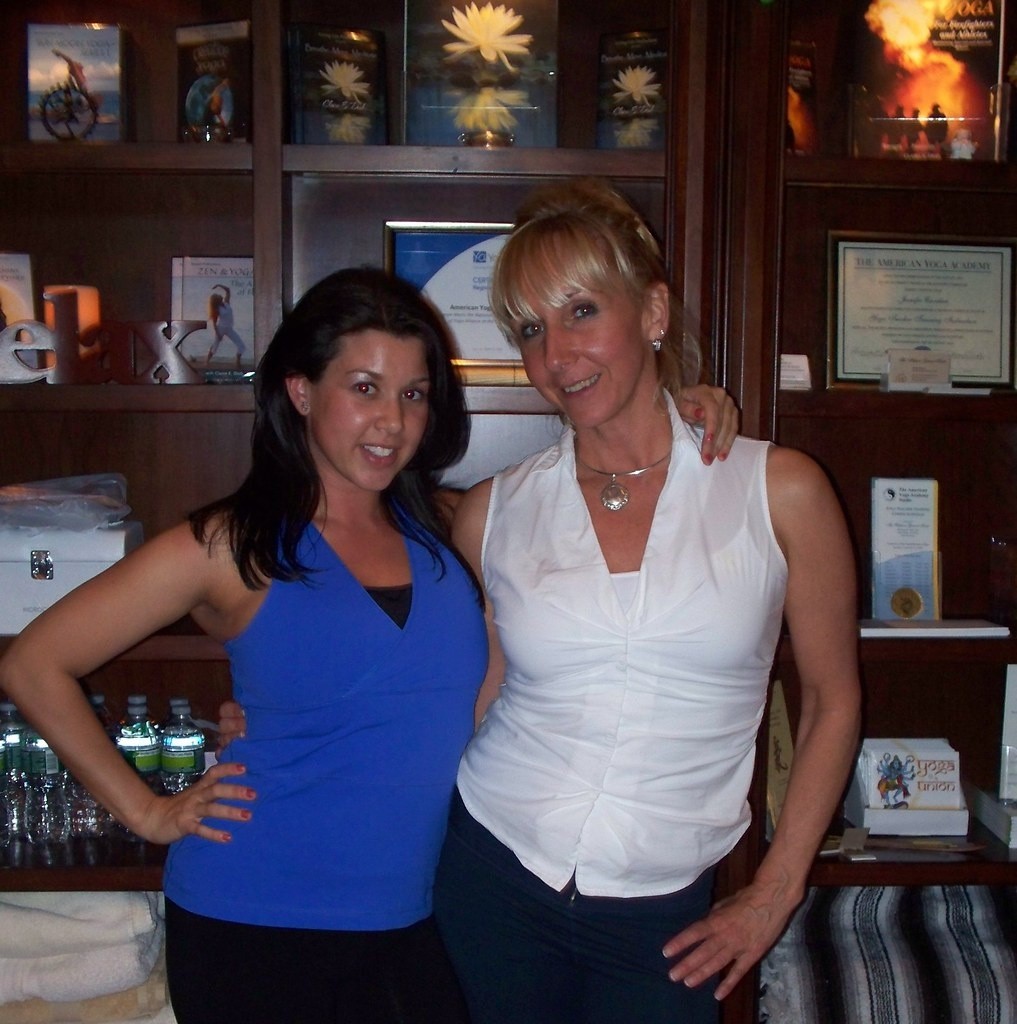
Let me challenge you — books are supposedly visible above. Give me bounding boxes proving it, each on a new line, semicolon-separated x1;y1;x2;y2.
169;257;255;362
844;0;1004;157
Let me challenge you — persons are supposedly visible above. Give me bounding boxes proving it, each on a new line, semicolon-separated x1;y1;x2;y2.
202;281;245;363
219;170;865;1024
1;260;739;1024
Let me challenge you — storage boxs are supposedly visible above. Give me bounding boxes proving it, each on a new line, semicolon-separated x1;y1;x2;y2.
842;765;969;836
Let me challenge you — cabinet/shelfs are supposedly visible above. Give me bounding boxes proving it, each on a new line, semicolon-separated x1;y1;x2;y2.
0;0;1017;885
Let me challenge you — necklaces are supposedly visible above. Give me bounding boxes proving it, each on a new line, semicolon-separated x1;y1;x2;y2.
574;448;672;508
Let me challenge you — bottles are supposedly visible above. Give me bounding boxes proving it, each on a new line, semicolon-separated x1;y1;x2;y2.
0;694;206;845
